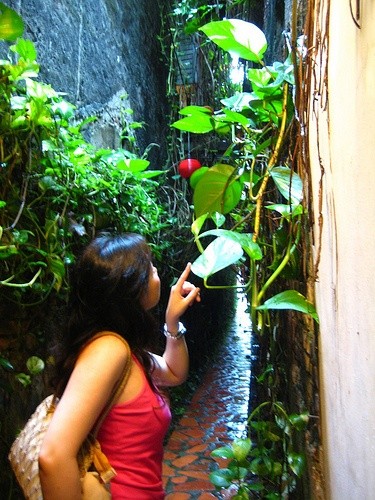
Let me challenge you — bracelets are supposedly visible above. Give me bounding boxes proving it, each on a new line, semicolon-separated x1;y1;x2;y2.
164;321;187;340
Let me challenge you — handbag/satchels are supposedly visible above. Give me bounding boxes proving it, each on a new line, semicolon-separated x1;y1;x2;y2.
8;331;133;500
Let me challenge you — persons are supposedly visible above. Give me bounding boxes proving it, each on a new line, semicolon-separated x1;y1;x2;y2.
37;231;201;500
